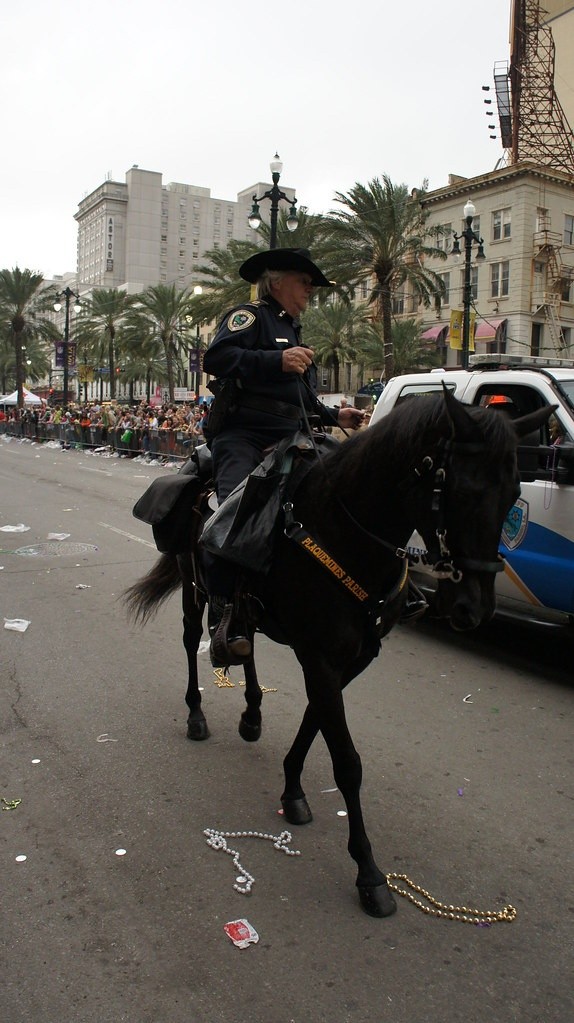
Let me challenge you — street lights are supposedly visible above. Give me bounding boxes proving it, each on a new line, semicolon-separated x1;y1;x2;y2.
53;287;81;405
449;197;486;369
248;150;299;249
185;286;207;406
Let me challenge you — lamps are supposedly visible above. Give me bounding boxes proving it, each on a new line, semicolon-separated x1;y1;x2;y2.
484;100;496;104
482;86;495;91
490;135;501;139
486;111;498;116
488;125;499;129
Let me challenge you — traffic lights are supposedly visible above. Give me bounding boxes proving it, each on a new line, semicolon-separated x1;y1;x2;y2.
115;367;125;373
93;367;102;372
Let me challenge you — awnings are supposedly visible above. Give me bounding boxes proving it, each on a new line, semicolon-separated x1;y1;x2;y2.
474;318;507;342
418;324;449;343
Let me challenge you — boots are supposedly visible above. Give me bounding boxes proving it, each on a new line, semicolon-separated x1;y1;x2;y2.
401;601;429;621
210;594;251;666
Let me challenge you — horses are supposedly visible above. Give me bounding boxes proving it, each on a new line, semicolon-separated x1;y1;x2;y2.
115;379;559;919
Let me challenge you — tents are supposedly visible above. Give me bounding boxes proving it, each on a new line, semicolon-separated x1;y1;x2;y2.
0;386;49;409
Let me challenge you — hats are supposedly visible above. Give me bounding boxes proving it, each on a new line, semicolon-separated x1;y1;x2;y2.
239;248;333;288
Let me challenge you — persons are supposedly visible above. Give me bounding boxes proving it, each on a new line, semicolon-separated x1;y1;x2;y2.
0;398;214;461
340;399;352;409
203;247;367;669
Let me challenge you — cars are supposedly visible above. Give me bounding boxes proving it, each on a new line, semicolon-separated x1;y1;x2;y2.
356;381;385;403
365;354;574;640
